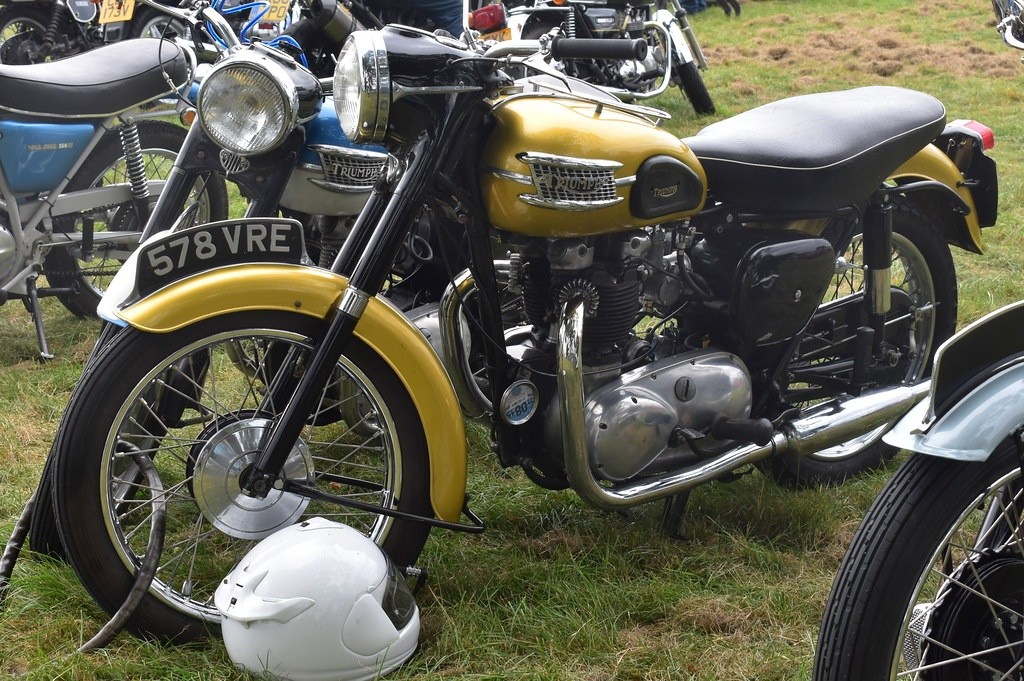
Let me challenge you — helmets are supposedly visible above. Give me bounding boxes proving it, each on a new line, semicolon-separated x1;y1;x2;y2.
214;516;420;681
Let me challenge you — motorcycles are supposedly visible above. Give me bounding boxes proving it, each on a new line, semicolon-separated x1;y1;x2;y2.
813;0;1023;678
0;2;1001;646
